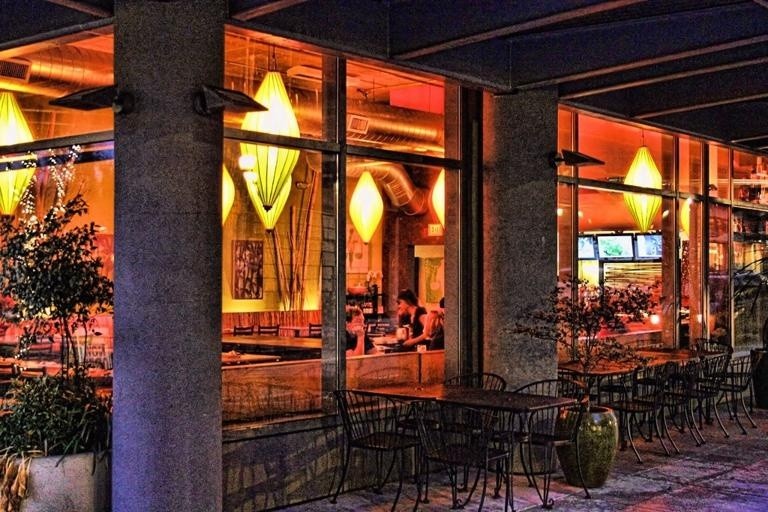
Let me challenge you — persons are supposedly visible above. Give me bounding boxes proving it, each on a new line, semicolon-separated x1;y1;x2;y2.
344;304;378;357
396;289;429;350
424;296;445;351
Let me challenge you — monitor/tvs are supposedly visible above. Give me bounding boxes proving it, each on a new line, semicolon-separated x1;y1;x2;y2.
596;233;635;262
635;231;662;262
578;234;596;260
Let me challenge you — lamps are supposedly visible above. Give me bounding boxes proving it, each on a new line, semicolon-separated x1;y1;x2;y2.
0;87;37;217
349;169;384;245
239;70;302;212
551;147;604;169
196;84;268;117
622;146;663;235
431;167;445;233
223;162;237;232
49;81;132;116
679;197;692;237
243;169;295;233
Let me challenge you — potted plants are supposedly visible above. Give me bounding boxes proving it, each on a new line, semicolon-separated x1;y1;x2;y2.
511;275;672;488
727;204;768;412
1;190;114;511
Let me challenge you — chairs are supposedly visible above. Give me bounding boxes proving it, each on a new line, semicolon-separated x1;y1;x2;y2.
559;338;766;465
221;311;445;363
2;313;115;424
329;372;593;510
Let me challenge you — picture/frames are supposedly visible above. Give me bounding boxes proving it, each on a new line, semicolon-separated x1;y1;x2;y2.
230;240;265;299
